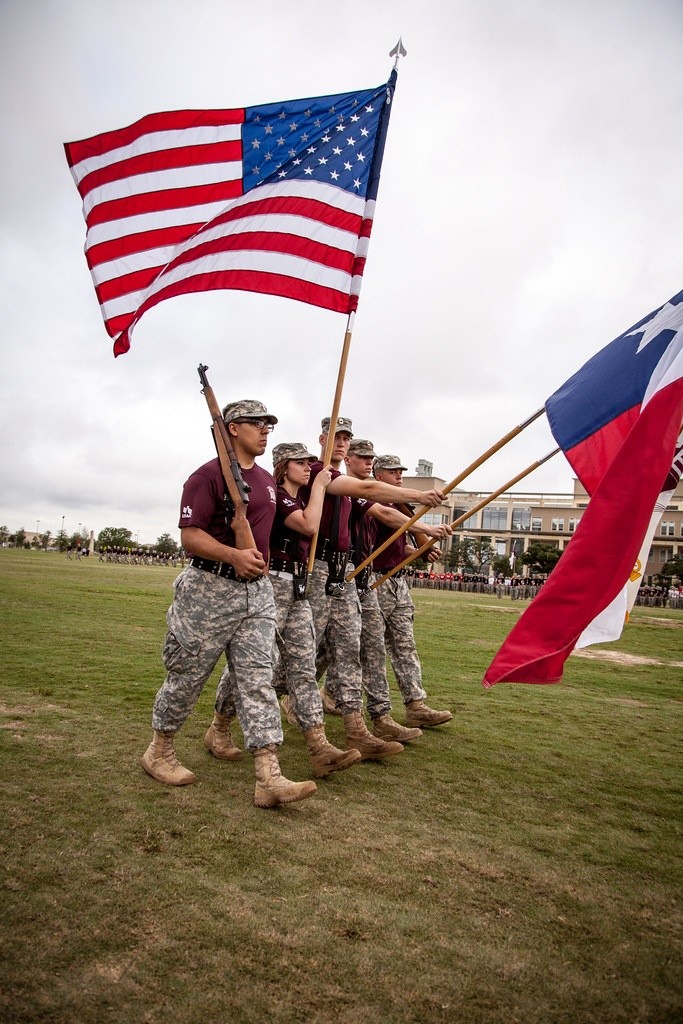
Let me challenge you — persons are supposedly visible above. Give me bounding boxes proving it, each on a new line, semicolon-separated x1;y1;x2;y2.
318;456;454;727
66;543;90;562
271;416;450;762
142;400;317;807
201;444;360;776
407;565;683;611
97;546;191;568
280;438;454;740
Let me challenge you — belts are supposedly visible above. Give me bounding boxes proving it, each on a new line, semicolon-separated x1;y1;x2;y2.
268;559;306;575
188;555;264;583
371;567;403;578
313;548;350;563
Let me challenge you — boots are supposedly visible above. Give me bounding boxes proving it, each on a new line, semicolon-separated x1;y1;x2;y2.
304;722;361;778
405;698;453;728
318;686;345;715
343;712;403;758
204;710;243;761
252;744;316;807
140;730;195;785
280;696;295;723
372;714;423;742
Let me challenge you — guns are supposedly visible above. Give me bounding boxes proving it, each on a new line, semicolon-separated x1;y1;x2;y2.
196;361;263;581
397;500;436;563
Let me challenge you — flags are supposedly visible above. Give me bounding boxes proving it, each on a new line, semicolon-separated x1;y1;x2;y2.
62;69;398;358
575;424;683;647
482;291;683;688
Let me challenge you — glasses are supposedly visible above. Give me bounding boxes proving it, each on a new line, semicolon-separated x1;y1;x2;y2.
233;419;274;432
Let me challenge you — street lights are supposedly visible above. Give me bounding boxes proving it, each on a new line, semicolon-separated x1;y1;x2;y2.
61;516;65;540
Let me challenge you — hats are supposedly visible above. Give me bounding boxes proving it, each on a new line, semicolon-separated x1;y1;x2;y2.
372;455;408;471
222;400;277;426
272;442;317;467
350;438;379;458
320;417;353;436
